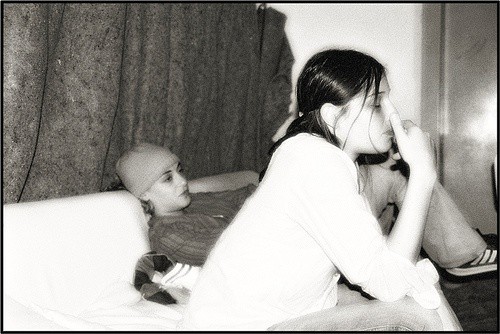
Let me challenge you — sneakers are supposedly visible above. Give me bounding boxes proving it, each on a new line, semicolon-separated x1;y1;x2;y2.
446;247;499;276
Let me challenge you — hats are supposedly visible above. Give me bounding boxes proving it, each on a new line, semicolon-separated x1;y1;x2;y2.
116;143;180;200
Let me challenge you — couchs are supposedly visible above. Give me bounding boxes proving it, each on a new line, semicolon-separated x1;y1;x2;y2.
4;170;260;331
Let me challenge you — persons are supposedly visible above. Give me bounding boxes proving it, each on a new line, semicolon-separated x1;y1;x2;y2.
115;143;500;276
186;49;463;333
